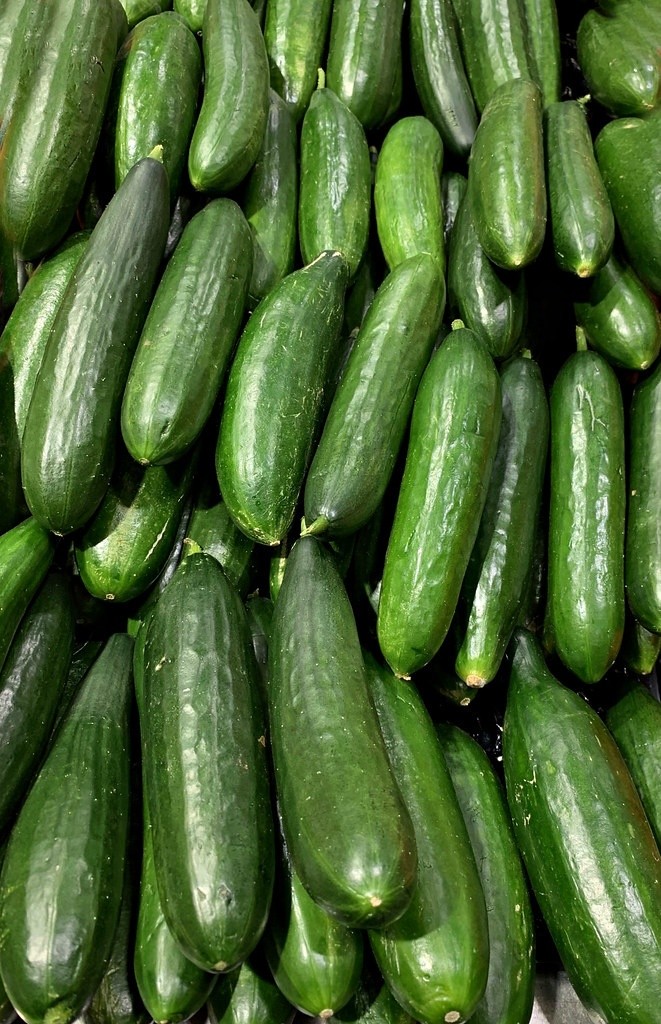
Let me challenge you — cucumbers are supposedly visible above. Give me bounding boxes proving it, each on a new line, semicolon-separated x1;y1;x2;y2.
0;0;661;1024
